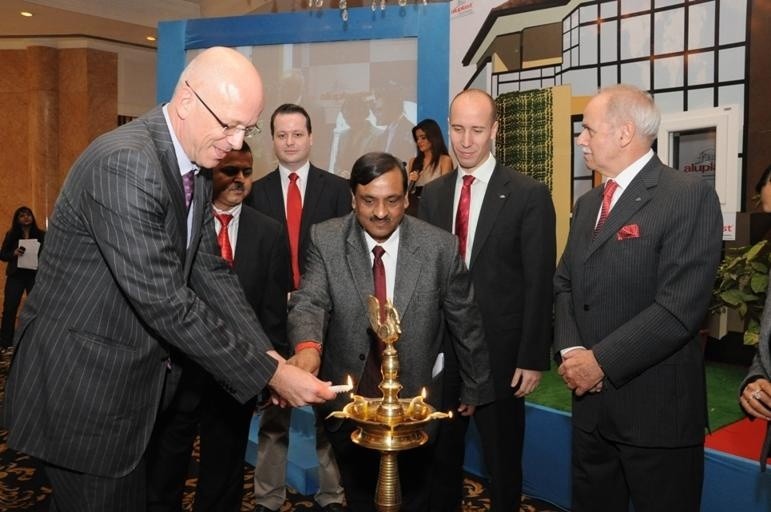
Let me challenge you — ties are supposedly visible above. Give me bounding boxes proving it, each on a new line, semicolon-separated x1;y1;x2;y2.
368;246;386;373
215;214;232;267
286;173;301;289
182;169;193;207
592;179;618;242
454;175;474;261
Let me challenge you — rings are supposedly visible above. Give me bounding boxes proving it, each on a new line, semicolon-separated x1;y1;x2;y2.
746;397;753;403
752;388;761;399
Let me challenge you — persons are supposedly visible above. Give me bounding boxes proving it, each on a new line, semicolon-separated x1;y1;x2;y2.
0;206;47;355
143;138;290;512
287;153;495;511
416;91;556;512
407;119;452;217
552;84;724;512
737;165;771;511
3;46;338;512
240;102;353;292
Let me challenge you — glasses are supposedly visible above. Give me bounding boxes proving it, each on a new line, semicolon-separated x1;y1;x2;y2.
184;81;260;139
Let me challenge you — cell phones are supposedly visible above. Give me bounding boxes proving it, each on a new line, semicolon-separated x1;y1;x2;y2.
19;246;25;254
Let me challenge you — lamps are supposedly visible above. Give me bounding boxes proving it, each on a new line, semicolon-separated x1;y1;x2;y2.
324;295;455;511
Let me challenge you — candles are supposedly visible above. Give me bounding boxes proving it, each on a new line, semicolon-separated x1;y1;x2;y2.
325;376;354;392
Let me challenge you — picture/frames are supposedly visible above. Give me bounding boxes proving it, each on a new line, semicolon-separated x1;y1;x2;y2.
655;104;738;213
